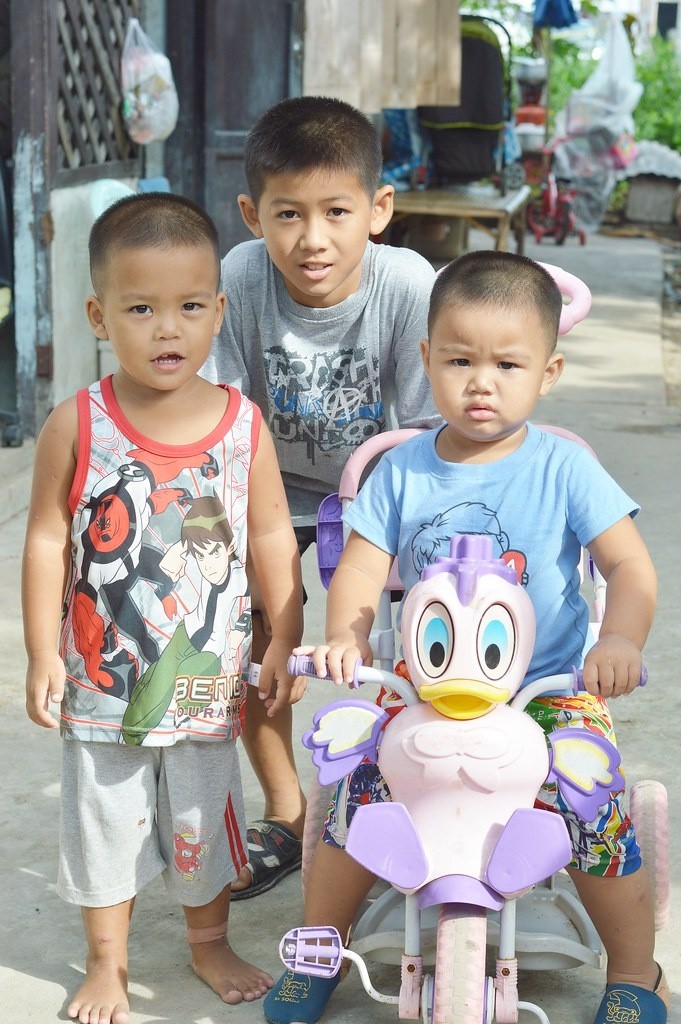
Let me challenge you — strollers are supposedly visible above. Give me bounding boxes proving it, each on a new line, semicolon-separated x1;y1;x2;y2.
415;12;527;198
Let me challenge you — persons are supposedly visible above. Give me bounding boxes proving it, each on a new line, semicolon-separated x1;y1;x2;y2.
260;250;669;1024
213;96;438;901
26;192;304;1024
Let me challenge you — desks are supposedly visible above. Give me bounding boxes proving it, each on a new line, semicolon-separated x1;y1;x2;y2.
394;181;530;255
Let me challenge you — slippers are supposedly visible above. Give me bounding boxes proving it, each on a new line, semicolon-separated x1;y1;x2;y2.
230;819;304;900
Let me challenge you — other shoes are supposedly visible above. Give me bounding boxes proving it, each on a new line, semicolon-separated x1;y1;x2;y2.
594;961;670;1024
263;959;352;1024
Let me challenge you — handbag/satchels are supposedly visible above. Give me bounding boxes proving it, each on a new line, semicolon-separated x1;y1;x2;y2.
120;17;179;145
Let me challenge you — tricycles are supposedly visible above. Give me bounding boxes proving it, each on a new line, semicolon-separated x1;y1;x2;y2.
529;134;589;248
280;253;671;1024
